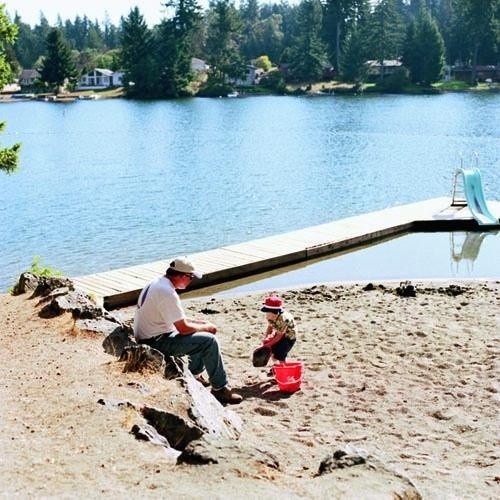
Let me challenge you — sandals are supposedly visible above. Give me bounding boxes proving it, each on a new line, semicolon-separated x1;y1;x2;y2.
267;369;276;377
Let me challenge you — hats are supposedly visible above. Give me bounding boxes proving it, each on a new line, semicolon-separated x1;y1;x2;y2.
169;256;202;279
260;297;284;313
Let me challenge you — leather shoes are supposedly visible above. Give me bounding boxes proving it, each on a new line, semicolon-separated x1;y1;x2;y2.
210;387;243;404
196;375;210;387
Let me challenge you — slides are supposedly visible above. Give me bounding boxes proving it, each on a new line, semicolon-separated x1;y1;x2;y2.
457;167;498;225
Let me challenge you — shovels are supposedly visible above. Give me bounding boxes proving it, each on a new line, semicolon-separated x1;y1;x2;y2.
253;341;273;367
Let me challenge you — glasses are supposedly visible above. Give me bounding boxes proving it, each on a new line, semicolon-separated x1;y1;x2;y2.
185;275;195;280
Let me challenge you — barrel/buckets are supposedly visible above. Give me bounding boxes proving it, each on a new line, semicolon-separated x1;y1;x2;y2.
272;362;305;392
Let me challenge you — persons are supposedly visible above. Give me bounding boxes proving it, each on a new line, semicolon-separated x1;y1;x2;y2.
261;297;297;365
134;258;243;404
